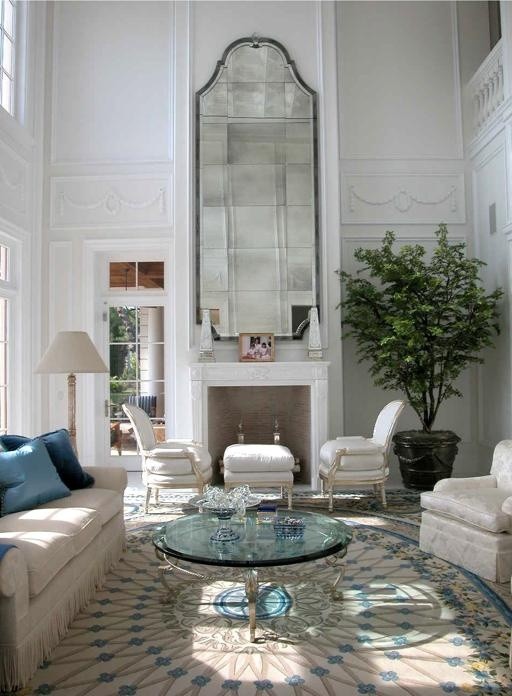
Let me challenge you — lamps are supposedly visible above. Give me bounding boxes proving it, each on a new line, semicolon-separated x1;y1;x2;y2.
33;331;110;460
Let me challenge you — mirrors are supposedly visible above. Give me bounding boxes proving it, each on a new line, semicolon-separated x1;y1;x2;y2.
195;33;320;340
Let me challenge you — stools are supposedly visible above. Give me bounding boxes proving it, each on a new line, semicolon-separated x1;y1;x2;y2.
224;443;294;510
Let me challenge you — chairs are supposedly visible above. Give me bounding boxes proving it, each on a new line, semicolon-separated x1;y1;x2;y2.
122;403;213;514
319;399;406;514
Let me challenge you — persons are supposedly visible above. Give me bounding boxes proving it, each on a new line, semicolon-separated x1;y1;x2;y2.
248;338;271;359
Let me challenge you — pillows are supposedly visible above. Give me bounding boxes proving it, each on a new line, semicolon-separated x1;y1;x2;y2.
319;439;384;471
146;443;211;475
0;437;72;519
1;426;95;491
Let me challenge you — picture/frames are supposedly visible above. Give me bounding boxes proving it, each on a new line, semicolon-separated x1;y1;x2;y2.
239;333;276;362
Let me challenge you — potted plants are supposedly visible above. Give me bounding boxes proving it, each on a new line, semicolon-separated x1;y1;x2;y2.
334;222;505;491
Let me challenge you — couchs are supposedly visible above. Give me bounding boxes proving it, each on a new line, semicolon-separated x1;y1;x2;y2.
419;440;512;584
0;466;128;693
